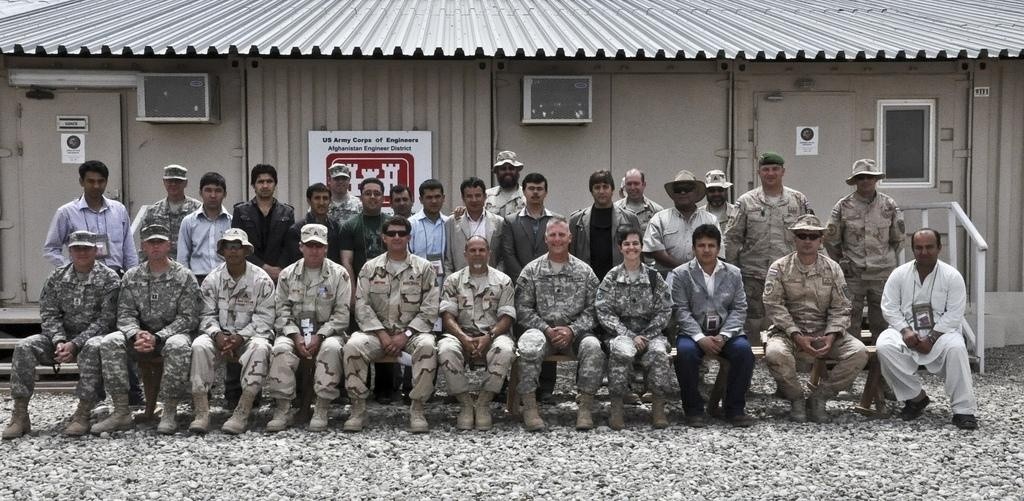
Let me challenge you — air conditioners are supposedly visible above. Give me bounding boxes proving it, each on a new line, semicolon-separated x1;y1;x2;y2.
521;75;593;123
136;73;221;125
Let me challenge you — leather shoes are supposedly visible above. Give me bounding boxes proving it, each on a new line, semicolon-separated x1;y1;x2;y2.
952;414;977;430
727;411;755;427
900;396;929;421
684;406;703;427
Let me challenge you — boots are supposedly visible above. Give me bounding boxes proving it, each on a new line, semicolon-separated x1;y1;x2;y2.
90;392;133;433
651;396;668;429
522;393;545;431
309;398;329;431
410;400;429;432
2;403;31;438
344;399;369;431
66;400;91;435
457;393;473;430
789;397;807;421
157;398;177;434
809;394;829;424
189;392;210;432
475;393;493;431
576;394;594;429
222;390;254;434
267;398;295;432
609;397;626;430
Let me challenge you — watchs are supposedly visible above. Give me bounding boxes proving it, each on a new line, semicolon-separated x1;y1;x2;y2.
404;327;412;337
487;328;496;338
926;333;938;345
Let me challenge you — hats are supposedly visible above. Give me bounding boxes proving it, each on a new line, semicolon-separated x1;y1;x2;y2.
300;224;329;245
328;163;351;178
142;223;171;241
846;159;885;185
664;170;706;202
68;231;97;247
493;150;524;170
217;228;254;257
162;164;188;181
704;169;733;188
759;152;785;165
787;213;831;231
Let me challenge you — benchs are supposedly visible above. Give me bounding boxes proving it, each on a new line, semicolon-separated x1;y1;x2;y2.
67;346;978;419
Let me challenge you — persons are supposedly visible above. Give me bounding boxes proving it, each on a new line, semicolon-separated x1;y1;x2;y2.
764;214;868;423
877;228;980;429
175;173;234;286
406;179;466;402
723;153;818;360
515;217;601;433
595;224;675;429
2;230;121;438
190;229;276;434
321;164;362;226
342;216;440;432
614;170;664;402
290;181;343;268
267;224;352;434
503;171;568;401
91;224;199;436
700;168;740;259
570;169;643;404
444;175;507;400
142;164;204;271
338;177;396;403
823;158;906;399
479;150;531;221
643;171;724;402
671;225;755;426
44;161;138;401
232;163;295;280
439;236;517;430
390;185;414;224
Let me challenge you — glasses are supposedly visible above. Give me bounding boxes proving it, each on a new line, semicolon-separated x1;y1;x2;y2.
796;233;821;239
674;188;693;194
710;188;723;193
224;242;241;249
385;230;407;237
306;242;323;248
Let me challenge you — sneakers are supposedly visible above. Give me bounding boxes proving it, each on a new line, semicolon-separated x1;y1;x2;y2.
129;387;161;408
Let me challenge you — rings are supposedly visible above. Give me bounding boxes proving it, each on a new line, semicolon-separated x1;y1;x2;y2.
638;347;639;350
563;340;567;344
